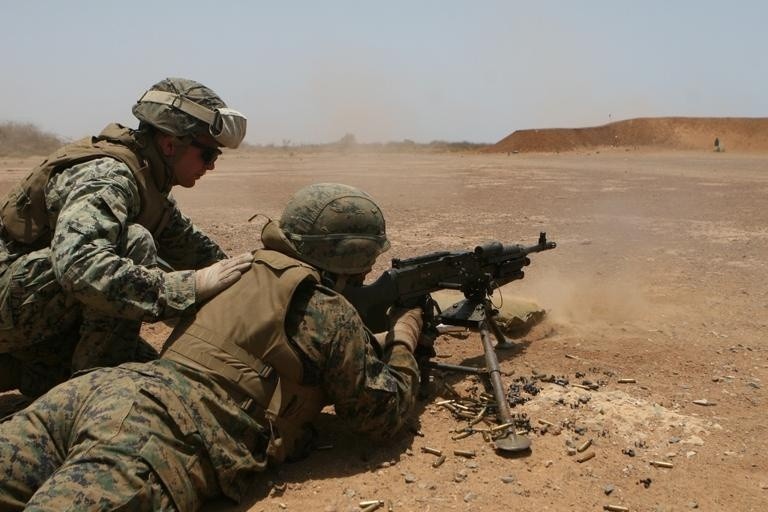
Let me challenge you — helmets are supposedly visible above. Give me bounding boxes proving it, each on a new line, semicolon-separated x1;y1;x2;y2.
133;77;248;149
278;182;391;275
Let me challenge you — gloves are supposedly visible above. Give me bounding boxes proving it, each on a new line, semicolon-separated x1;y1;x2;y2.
383;303;425;355
192;251;255;303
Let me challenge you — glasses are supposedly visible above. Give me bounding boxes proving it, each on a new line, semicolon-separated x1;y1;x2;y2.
190;140;222;164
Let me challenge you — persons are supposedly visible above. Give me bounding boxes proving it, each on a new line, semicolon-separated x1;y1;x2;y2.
0;182;423;512
0;76;259;378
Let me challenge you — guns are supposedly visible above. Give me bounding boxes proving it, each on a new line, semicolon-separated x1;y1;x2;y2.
343;232;557;451
